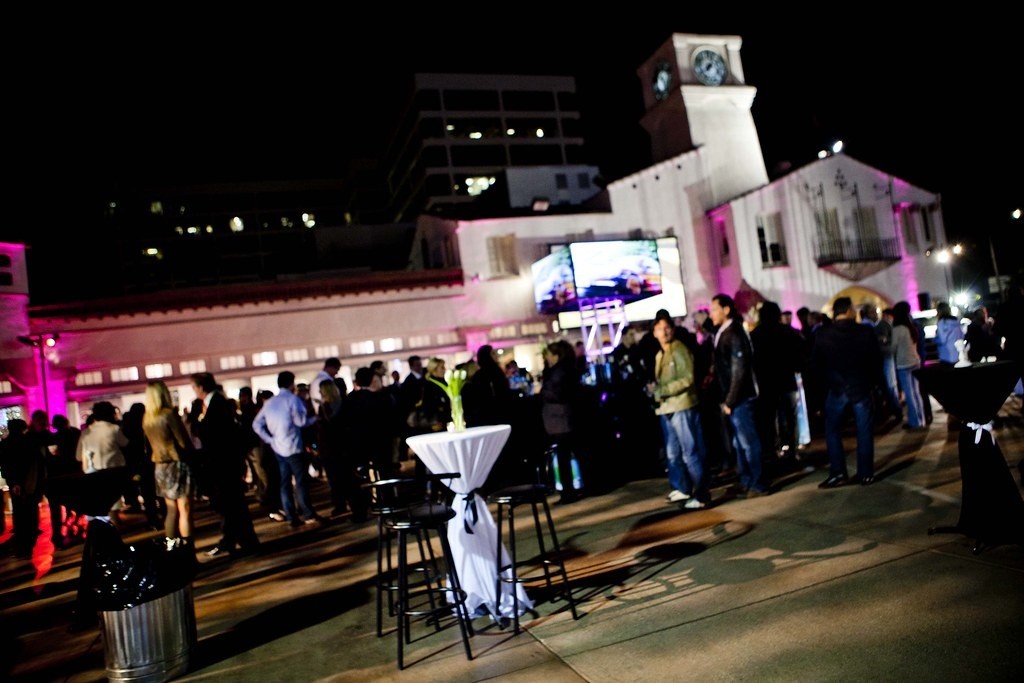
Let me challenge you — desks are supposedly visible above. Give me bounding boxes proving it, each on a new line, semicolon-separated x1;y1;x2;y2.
910;356;1023;557
406;424;540;629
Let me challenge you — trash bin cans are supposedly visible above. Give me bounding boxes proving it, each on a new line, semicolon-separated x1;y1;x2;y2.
87;536;194;683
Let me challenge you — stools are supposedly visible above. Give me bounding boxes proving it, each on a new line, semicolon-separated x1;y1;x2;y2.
487;444;577;636
353;463;475;669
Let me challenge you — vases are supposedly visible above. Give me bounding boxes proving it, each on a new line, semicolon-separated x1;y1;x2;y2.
449;395;466;432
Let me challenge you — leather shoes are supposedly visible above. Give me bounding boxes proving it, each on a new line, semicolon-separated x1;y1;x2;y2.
851;473;875;484
818;473;848;488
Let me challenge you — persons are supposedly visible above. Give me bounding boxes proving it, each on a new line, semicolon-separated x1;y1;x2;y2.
0;379;210;573
607;292;930;508
386;338;602;511
929;274;1024;364
185;358;383;553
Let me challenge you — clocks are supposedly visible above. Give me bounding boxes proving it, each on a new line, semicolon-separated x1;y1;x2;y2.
691;45;729;86
651;57;675;102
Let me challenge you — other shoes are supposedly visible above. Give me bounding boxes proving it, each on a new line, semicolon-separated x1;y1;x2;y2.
204;547;232;556
305;514;324;525
684;499;706;508
289;520;304;527
230;548;262;563
723;485;747;494
666;489;691;503
267;512;285;521
736;487;772;498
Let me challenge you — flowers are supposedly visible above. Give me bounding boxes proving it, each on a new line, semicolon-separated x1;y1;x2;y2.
429;367;467;415
